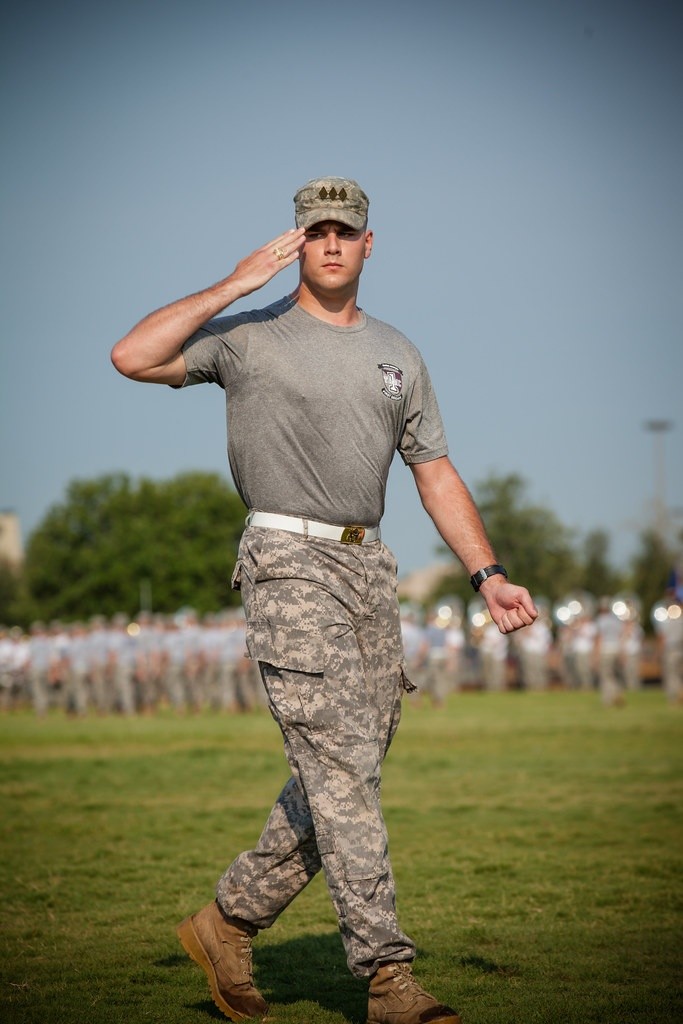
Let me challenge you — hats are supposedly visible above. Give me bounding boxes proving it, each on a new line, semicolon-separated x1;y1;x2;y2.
292;177;371;231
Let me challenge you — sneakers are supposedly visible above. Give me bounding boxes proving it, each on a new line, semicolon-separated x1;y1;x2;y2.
367;962;460;1024
179;899;269;1022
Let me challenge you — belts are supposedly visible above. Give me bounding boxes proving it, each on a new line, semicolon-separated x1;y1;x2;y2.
250;512;380;545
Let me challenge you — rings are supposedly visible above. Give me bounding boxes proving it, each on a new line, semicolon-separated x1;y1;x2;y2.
273;248;284;259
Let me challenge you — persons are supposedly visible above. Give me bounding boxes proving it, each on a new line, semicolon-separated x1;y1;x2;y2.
1;592;683;715
110;176;539;1023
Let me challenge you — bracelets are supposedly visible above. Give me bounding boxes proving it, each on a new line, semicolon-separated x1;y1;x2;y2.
471;565;507;592
